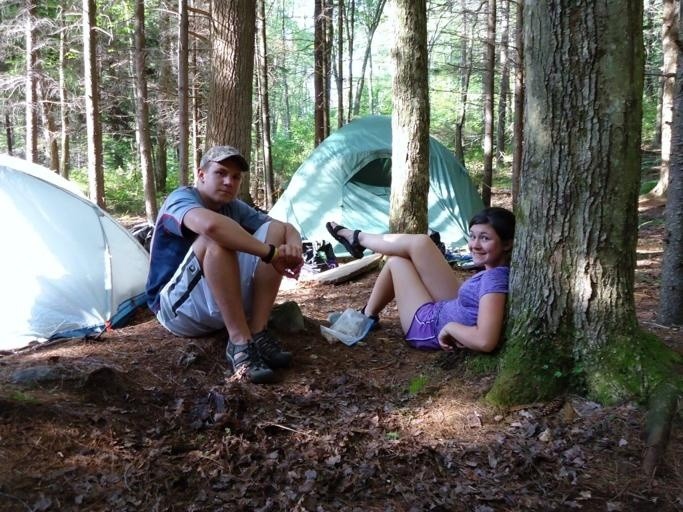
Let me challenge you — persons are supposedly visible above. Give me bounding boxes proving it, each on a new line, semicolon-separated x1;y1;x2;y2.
144;143;306;384
326;206;515;352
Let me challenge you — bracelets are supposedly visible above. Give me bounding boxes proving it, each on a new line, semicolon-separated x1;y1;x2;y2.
260;239;280;265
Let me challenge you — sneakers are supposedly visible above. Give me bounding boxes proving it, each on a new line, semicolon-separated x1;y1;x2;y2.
225;340;275;384
251;328;293;366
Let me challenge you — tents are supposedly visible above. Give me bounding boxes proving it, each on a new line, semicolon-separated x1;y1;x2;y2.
0;154;151;350
266;115;486;289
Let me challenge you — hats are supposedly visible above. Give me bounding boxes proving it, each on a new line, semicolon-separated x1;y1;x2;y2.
200;146;249;173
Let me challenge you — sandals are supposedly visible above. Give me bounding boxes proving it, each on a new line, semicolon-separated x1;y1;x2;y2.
327;222;367;259
357;305;380;331
